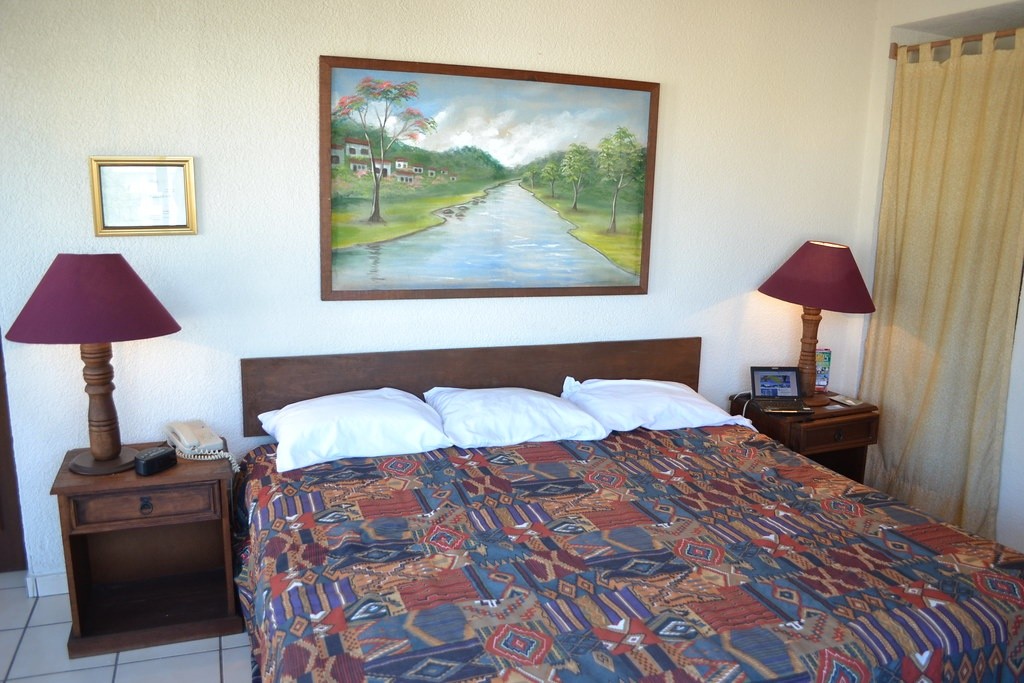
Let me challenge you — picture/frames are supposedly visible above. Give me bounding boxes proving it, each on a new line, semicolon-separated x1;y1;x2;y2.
317;54;660;302
89;154;198;236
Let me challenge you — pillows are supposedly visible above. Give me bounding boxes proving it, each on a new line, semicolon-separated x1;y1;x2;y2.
257;386;453;474
561;376;752;432
422;387;607;449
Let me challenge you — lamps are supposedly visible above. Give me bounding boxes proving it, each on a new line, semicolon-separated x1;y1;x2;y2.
758;241;876;406
6;254;183;479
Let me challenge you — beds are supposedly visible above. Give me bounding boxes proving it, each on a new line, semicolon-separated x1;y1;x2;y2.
236;337;1024;683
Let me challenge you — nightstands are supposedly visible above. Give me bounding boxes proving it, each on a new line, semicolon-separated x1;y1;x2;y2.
729;390;881;486
50;440;246;659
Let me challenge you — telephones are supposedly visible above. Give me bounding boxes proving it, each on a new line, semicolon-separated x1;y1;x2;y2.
162;417;224;454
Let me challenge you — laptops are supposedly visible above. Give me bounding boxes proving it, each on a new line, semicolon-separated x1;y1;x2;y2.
750;366;814;414
